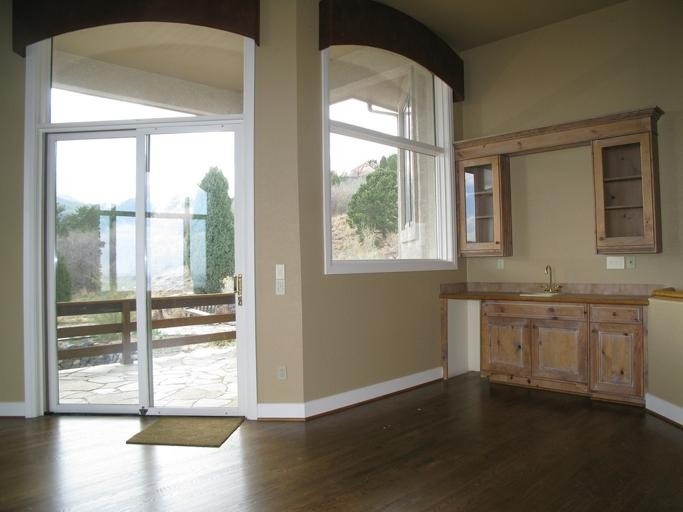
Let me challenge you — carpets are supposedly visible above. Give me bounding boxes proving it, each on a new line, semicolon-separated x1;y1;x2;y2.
126;415;247;447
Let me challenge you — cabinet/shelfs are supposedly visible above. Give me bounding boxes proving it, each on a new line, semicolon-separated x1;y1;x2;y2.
456;155;512;257
480;301;645;406
591;131;664;256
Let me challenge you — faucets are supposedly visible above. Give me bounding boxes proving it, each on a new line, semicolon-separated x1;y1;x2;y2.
545;264;553;291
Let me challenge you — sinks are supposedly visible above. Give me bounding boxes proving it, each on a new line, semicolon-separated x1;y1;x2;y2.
519;292;559;298
